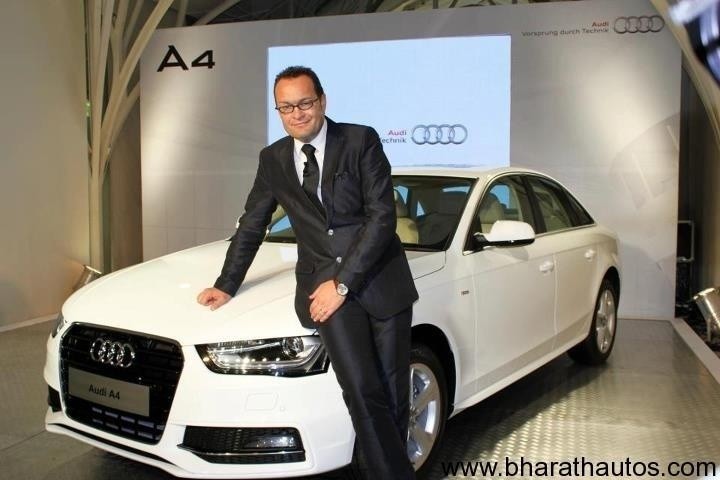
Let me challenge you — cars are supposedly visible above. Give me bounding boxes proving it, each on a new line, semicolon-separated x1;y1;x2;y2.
41;163;621;479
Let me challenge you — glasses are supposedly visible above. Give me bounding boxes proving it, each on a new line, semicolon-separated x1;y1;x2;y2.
275;98;318;113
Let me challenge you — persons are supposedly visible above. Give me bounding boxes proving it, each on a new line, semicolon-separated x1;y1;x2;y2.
196;66;422;479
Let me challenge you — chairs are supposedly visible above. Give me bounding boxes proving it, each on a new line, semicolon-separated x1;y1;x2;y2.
394;189;569;243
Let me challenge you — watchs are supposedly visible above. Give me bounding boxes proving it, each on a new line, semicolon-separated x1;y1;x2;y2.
333;278;348;296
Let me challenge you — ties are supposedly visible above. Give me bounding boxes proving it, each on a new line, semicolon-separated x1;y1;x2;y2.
302;144;326;218
